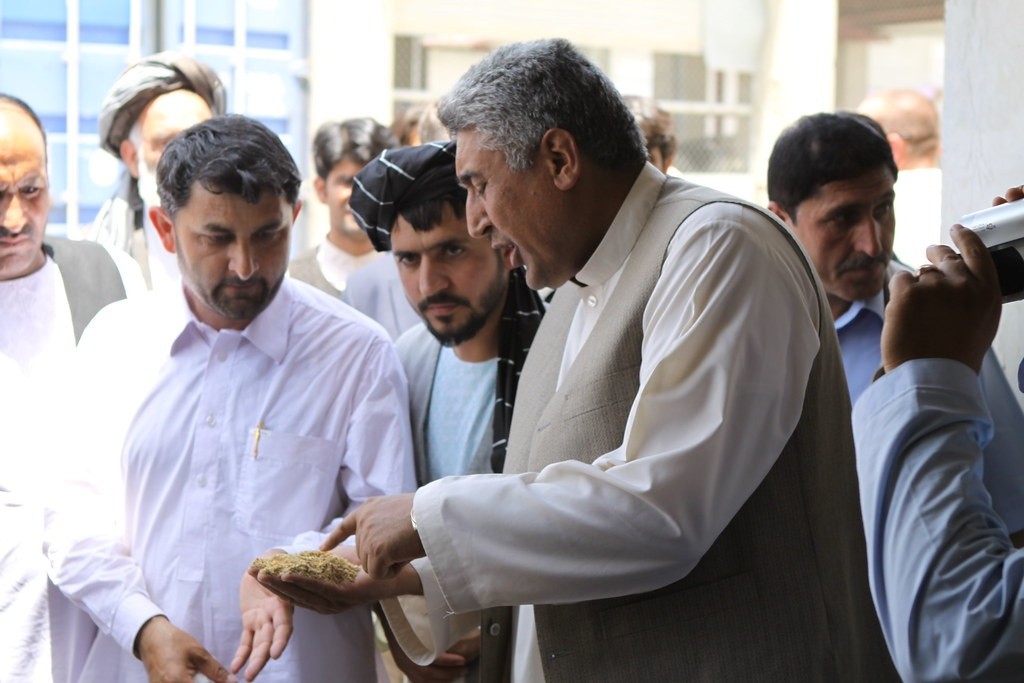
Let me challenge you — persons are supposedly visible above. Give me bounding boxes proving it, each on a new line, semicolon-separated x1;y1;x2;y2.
0;54;1024;683
249;39;907;683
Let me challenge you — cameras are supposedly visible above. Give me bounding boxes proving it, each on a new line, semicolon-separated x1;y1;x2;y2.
959;198;1024;304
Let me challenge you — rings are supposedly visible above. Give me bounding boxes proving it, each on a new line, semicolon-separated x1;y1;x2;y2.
914;265;945;281
